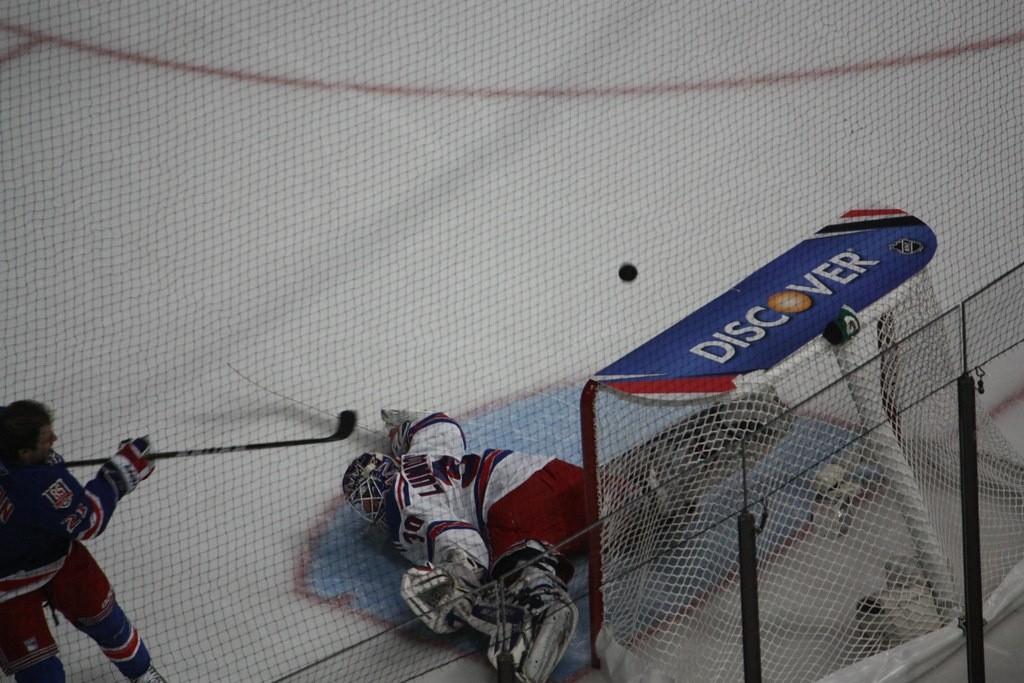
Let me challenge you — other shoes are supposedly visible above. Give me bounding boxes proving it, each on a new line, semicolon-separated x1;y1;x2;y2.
511;598;579;683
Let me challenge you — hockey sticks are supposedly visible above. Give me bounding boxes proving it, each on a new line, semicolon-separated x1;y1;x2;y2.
64;408;359;469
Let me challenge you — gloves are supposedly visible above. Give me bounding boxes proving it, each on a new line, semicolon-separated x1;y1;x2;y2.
99;434;156;502
400;566;472;634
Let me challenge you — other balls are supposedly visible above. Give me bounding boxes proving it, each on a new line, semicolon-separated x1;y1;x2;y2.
619;264;638;281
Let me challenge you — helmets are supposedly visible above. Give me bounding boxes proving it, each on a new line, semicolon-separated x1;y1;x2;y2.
342;452;400;531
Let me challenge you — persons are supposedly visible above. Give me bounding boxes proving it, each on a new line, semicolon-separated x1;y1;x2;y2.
343;388;793;683
0;400;167;683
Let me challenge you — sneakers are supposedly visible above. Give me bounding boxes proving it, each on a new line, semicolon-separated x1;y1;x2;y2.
130;665;168;683
